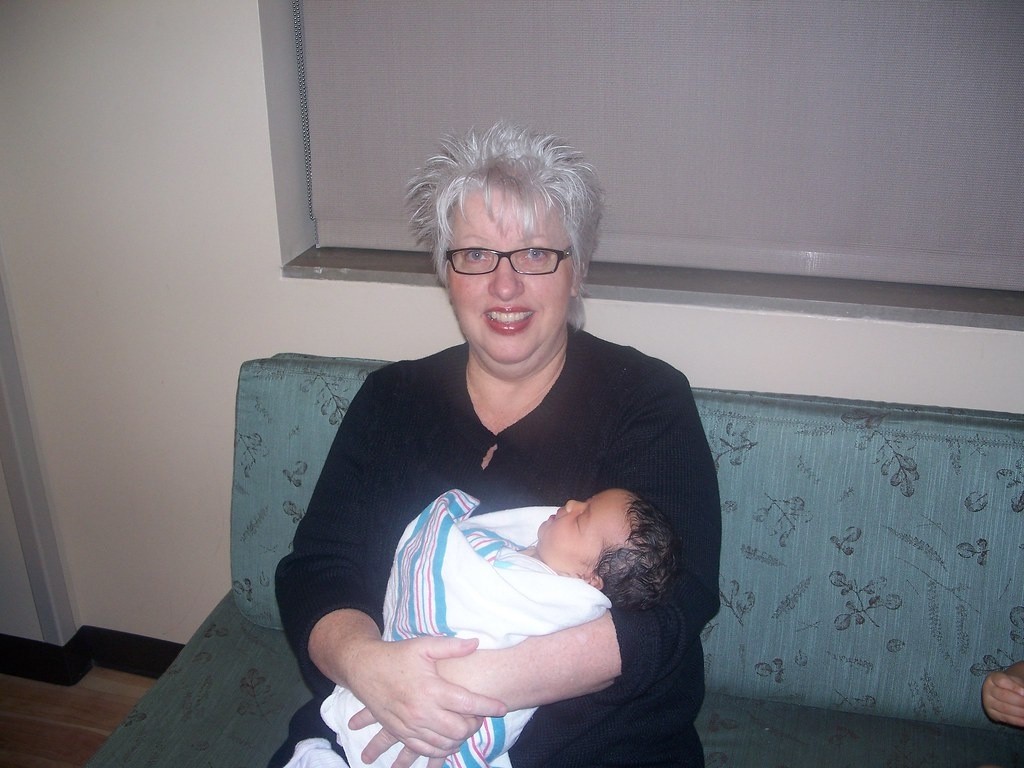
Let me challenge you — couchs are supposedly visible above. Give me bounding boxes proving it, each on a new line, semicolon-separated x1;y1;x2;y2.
87;353;1024;768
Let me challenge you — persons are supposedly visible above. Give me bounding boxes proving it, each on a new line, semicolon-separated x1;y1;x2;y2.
983;660;1024;726
265;119;722;768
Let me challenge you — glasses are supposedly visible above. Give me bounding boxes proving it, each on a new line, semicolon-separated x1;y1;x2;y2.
446;246;573;275
